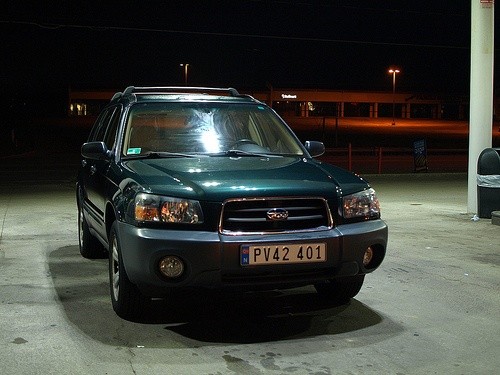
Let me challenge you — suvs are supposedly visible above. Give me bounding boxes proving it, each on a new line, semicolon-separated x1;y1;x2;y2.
75;86;389;325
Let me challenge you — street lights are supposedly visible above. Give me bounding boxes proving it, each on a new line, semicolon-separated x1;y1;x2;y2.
389;69;400;126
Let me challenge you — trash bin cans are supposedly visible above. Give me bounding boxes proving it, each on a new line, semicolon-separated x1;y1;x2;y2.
476;148;500;219
